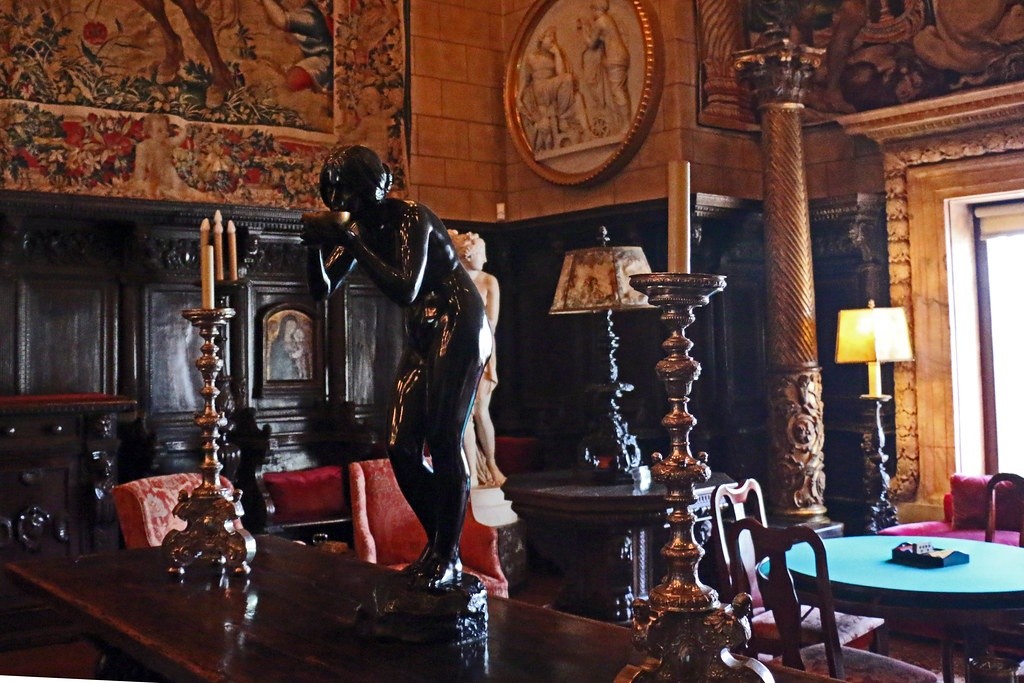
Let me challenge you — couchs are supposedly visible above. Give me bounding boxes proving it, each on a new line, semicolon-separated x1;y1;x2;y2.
877;493;1024;654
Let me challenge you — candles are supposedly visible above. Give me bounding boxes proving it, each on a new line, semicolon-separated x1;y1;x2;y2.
214;210;223;281
202;245;215;310
228;220;239;281
668;159;691;274
200;218;209;245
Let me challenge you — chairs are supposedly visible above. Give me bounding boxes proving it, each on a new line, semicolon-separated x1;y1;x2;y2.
711;478;886;648
349;460;508;600
112;473;235;549
726;519;936;683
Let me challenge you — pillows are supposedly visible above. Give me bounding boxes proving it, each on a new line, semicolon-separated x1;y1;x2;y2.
951;474;1020;531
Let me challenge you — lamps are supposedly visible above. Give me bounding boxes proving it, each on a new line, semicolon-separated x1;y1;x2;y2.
549;225;661;486
834;300;917;536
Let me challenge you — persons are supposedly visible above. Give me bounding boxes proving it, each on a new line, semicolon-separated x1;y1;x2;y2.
448;230;508;488
517;26;573;150
305;143;494;591
578;0;632;135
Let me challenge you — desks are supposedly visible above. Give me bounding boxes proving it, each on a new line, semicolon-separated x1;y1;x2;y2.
756;534;1024;683
0;535;843;683
508;471;733;628
